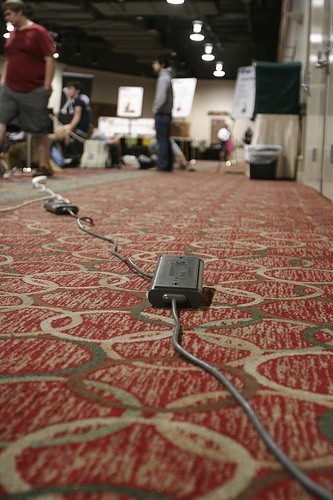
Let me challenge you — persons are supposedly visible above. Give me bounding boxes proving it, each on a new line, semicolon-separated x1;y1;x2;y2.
37;82;92;168
148;56;176;171
0;1;57;177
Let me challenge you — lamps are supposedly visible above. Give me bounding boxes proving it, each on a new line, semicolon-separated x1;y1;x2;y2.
213;60;227;77
201;42;217;61
190;19;204;43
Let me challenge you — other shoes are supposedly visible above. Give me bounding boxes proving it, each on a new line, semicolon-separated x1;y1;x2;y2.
32;168;54;176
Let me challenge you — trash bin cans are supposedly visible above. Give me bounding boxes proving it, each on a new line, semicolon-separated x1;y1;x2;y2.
245;145;282;180
